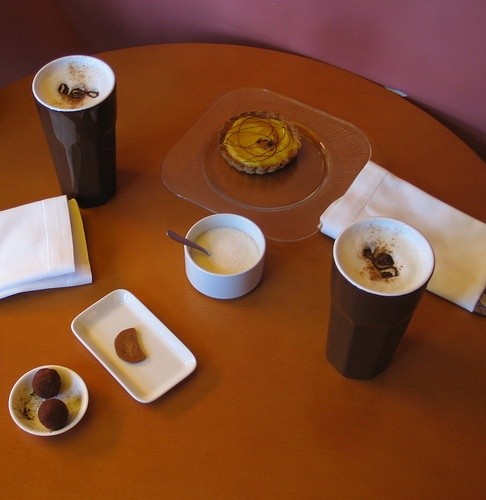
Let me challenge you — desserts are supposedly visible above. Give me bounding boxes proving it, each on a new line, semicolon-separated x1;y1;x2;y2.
218;110;303;174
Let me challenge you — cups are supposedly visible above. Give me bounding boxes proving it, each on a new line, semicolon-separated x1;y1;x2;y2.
325;217;436;380
32;54;118;209
183;213;266;300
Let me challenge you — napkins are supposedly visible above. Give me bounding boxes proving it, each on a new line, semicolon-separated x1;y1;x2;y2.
316;158;486;314
0;195;93;300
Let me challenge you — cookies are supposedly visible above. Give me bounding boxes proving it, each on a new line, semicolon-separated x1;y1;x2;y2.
114;328;146;363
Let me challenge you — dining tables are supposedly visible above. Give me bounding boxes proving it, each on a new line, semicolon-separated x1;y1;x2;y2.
0;41;486;500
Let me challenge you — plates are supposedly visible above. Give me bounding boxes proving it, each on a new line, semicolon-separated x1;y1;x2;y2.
70;289;196;403
160;87;373;244
8;365;89;436
